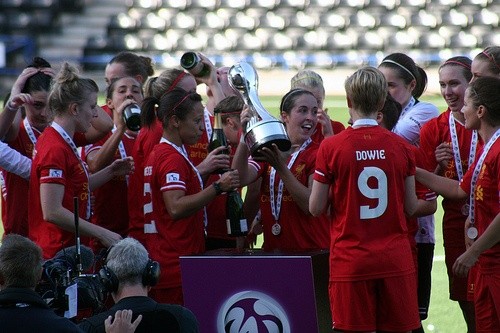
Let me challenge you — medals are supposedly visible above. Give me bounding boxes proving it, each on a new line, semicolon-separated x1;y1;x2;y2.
461;204;470;217
466;226;479;239
272;224;282;236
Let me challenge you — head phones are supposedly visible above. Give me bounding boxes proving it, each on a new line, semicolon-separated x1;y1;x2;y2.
98;243;160;290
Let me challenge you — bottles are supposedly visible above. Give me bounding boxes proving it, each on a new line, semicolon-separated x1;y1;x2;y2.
225;169;249;238
209;107;229;174
180;51;210;77
122;102;144;131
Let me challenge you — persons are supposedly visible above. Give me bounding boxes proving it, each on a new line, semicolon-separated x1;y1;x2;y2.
0;45;500;333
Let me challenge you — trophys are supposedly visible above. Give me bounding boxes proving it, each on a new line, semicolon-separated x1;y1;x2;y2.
228;61;291;158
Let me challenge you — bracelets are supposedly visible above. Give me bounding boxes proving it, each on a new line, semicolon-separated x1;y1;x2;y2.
211;182;223;197
7;100;19;112
